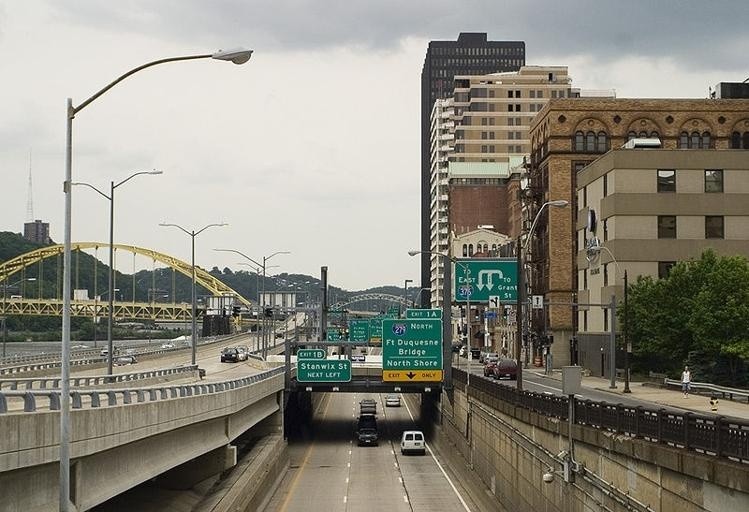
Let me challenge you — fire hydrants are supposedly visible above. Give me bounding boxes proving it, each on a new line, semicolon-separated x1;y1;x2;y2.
709;396;719;414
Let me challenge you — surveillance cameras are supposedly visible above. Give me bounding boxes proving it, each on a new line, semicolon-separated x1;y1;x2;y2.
543;473;553;483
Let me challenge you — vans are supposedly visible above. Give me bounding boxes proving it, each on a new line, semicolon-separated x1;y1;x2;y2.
400;431;426;456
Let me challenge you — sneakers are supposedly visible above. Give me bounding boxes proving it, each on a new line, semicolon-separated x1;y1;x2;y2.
681;393;689;399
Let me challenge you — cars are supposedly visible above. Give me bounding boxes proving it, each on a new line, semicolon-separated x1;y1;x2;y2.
385;395;401;406
356;415;378;445
359;398;377;413
451;340;480;358
484;353;517;380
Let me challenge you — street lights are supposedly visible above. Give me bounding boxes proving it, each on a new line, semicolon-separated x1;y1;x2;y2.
516;199;569;390
407;250;472;373
590;245;630;391
213;248;290;350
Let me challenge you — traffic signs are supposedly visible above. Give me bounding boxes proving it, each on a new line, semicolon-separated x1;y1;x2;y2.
350;317;381;344
381;308;444;382
296;349;352;383
327;330;340;341
453;257;526;305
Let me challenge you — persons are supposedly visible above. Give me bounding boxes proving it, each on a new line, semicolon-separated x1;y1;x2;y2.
680;365;692;399
